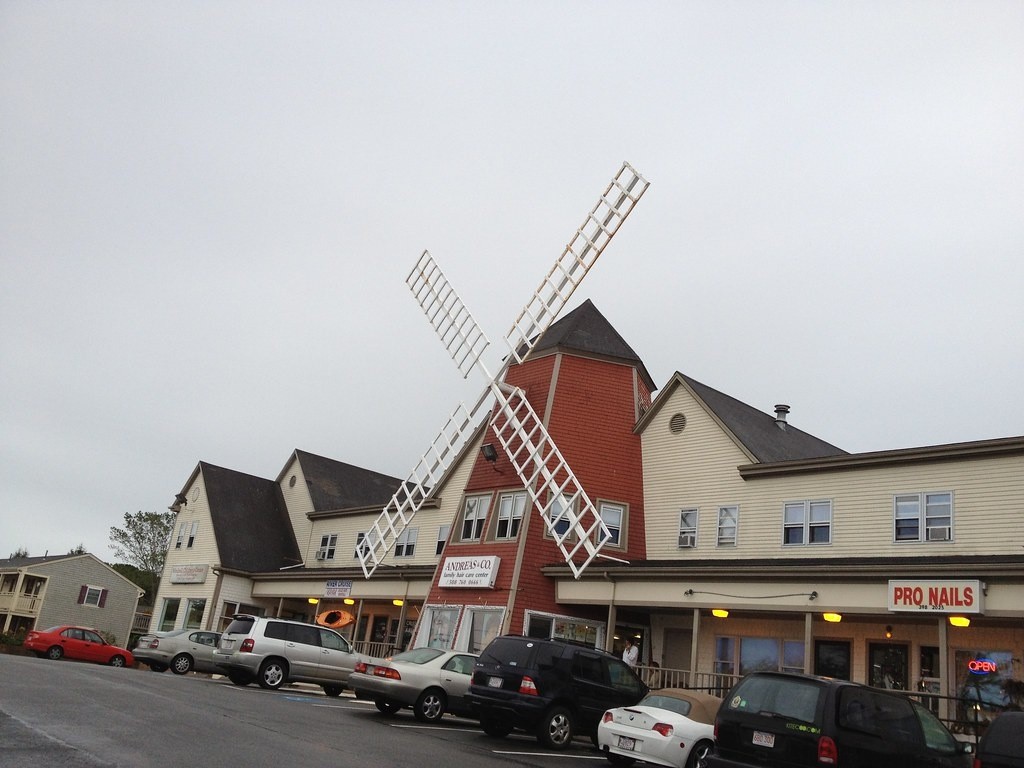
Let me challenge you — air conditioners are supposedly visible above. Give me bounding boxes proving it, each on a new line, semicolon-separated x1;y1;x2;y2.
316;551;326;560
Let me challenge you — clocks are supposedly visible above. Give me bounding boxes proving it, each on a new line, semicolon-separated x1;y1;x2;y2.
192;487;200;502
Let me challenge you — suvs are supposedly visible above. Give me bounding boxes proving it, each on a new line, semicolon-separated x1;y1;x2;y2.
700;670;976;768
973;712;1024;768
464;633;653;751
212;613;375;701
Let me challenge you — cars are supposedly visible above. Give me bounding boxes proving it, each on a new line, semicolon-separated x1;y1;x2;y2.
23;624;136;667
131;628;230;675
596;687;725;768
346;646;480;723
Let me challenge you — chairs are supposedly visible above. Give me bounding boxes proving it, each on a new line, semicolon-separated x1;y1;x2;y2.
205;639;213;646
446;660;456;672
198;638;204;644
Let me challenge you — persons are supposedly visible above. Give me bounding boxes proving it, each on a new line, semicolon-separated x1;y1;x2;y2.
622;638;640;674
643;661;660;688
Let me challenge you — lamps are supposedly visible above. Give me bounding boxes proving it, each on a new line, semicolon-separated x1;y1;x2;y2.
712;609;729;617
479;442;502;474
950;616;971;627
823;613;842;622
393;599;403;606
308;598;318;604
613;634;620;640
344;598;354;605
886;626;892;639
